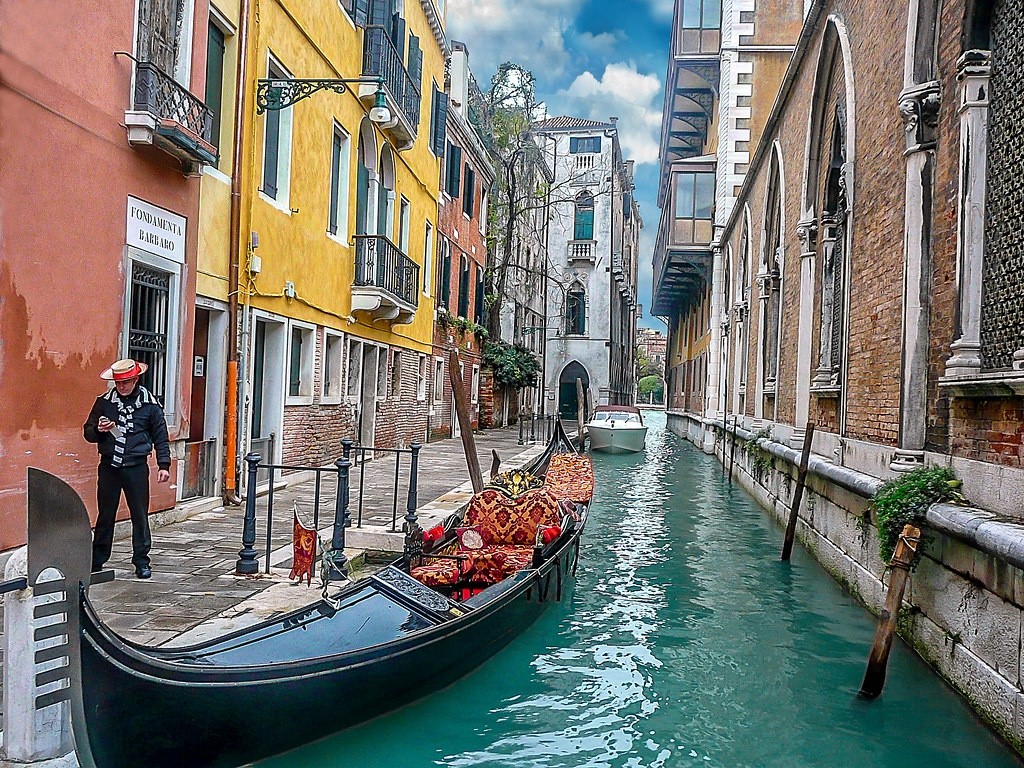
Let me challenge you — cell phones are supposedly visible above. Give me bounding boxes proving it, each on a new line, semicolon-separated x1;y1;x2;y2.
99;417;111;423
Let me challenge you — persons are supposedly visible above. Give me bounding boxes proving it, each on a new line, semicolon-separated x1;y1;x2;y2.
82;359;172;579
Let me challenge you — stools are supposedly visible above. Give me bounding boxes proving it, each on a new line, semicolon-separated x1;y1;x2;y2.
411;566;459;597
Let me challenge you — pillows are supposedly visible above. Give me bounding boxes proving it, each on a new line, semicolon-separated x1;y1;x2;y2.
451;524;488;550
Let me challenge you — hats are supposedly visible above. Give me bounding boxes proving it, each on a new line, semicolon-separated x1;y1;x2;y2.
100;359;148;381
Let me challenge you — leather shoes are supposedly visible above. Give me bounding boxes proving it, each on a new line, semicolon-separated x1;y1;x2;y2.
138;567;150;578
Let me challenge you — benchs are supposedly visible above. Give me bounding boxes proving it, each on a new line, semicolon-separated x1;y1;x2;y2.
410;469;573;600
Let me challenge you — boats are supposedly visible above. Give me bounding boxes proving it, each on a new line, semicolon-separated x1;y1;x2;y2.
27;414;594;768
585;405;650;455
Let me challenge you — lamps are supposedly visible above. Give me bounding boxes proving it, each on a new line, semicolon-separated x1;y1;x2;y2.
256;78;390;121
